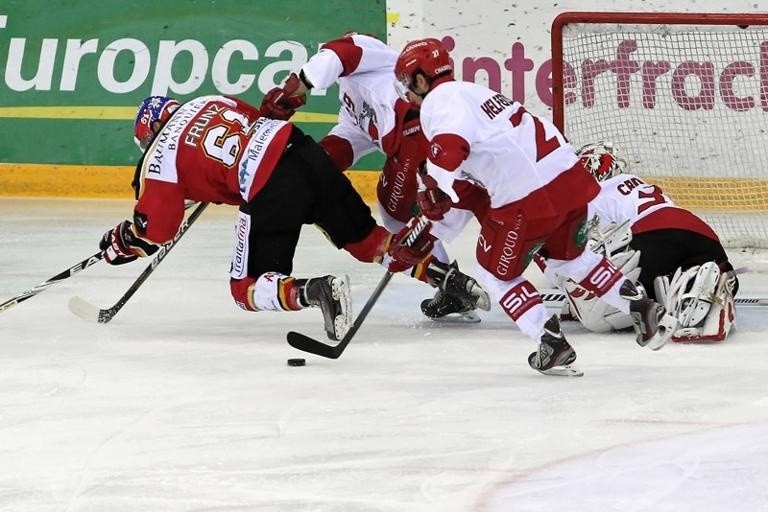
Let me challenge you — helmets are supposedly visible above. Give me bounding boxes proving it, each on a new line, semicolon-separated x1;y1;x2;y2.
133;96;180;154
394;39;454;103
575;141;622;182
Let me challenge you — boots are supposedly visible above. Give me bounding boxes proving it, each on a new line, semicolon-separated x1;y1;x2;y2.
297;276;340;342
529;315;576;370
619;259;720;346
421;260;481;317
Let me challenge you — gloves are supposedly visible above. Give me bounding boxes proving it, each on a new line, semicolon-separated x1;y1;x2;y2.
259;73;306;121
99;221;160;265
389;213;438;272
416;160;453;220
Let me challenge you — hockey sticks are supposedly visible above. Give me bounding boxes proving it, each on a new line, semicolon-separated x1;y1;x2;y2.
286;263;393;360
0;196;198;316
67;71;304;324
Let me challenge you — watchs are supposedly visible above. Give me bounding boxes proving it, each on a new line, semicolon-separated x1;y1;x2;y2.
255;32;486;326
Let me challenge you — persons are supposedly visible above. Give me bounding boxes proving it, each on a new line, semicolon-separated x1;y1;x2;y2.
392;37;679;374
531;141;740;352
96;94;491;341
